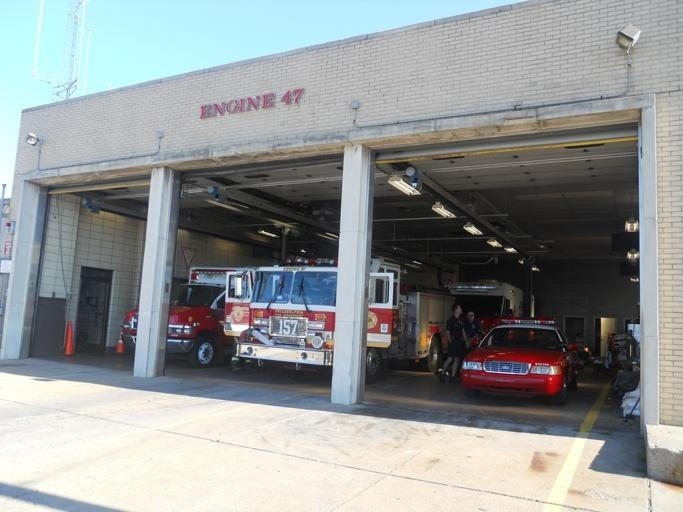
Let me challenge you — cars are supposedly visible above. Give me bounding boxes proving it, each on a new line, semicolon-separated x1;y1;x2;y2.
459;319;579;406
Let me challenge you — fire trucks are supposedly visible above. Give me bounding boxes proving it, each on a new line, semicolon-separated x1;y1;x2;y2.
122;266;241;369
223;248;536;385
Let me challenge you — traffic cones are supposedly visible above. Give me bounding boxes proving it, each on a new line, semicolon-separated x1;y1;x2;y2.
116;322;128;356
63;320;75;356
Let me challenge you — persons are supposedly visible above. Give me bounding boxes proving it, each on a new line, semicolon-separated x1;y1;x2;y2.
462;310;482;348
438;303;462;384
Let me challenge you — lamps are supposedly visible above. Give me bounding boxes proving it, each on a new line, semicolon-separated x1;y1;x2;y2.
25;133;38;145
463;221;504;248
388;175;421;196
432;201;456;219
617;24;641;51
